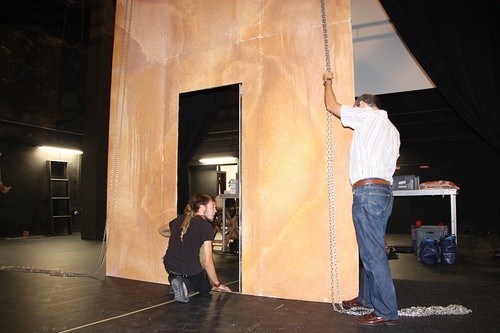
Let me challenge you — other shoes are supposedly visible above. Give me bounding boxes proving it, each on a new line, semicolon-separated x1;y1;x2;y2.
168;275;176;296
171;276;190;303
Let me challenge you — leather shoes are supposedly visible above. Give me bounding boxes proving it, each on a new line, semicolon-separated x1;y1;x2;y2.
354;311;401;325
341;297;363;309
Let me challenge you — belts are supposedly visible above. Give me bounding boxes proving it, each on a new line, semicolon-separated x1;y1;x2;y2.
353;179;390;189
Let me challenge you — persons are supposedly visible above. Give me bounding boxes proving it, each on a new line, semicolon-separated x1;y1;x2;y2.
0;180;10;194
158;193;232;303
322;71;401;326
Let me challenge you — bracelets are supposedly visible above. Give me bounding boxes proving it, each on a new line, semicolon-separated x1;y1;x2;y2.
215;281;221;288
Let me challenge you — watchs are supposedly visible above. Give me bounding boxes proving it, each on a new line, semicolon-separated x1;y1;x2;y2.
323;78;332;86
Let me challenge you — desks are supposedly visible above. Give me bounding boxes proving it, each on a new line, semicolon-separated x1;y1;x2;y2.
219;194;239;252
392;188;457;245
